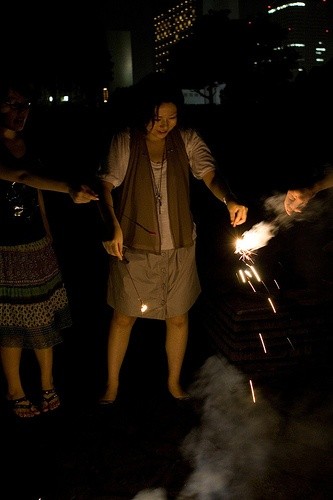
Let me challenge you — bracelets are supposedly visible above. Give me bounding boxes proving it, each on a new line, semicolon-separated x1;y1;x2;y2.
223;190;238;206
308;184;317;197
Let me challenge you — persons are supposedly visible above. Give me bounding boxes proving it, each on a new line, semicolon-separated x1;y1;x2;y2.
95;91;249;409
0;76;100;420
284;173;333;216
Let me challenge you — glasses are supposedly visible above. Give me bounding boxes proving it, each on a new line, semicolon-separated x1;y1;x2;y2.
3;102;31;111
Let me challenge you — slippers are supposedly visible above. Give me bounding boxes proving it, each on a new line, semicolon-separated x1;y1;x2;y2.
7;395;40;422
40;389;61;414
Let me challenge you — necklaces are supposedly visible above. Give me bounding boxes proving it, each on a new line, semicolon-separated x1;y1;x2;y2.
151;138;166;215
